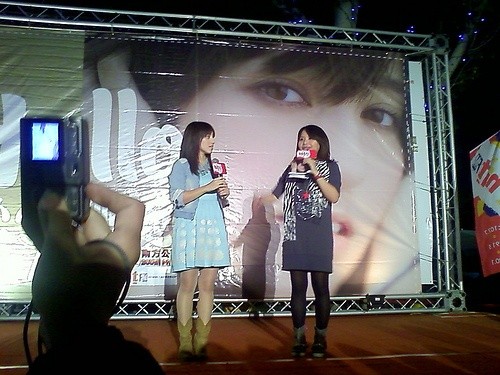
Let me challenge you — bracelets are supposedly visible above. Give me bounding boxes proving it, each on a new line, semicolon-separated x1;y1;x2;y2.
313;172;322;181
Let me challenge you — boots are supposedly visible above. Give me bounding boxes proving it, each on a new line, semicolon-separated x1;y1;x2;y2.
291;323;307;357
312;327;328;358
177;316;213;360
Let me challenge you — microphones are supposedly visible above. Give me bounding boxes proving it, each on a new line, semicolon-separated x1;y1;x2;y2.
296;148;317;177
212;159;229;208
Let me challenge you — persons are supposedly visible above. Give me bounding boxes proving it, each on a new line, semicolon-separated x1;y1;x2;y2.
80;30;415;299
253;123;342;359
26;181;167;375
167;120;231;364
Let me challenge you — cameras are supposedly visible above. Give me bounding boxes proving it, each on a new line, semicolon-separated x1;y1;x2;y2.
20;117;90;224
